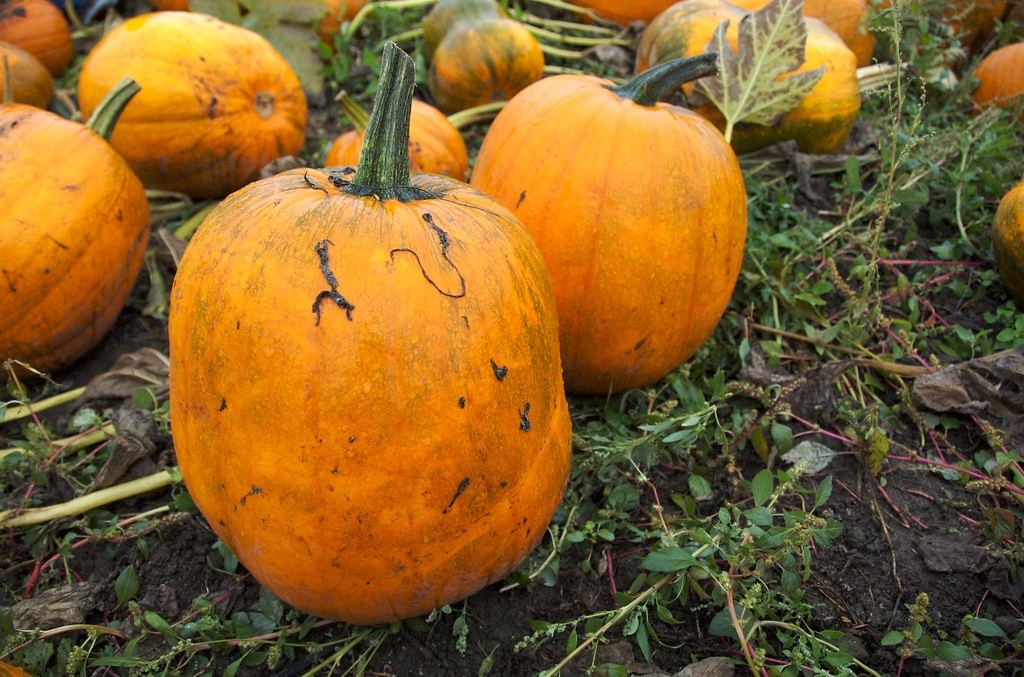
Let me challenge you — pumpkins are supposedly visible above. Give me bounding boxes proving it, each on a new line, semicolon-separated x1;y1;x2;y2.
0;0;1024;403
169;41;572;625
